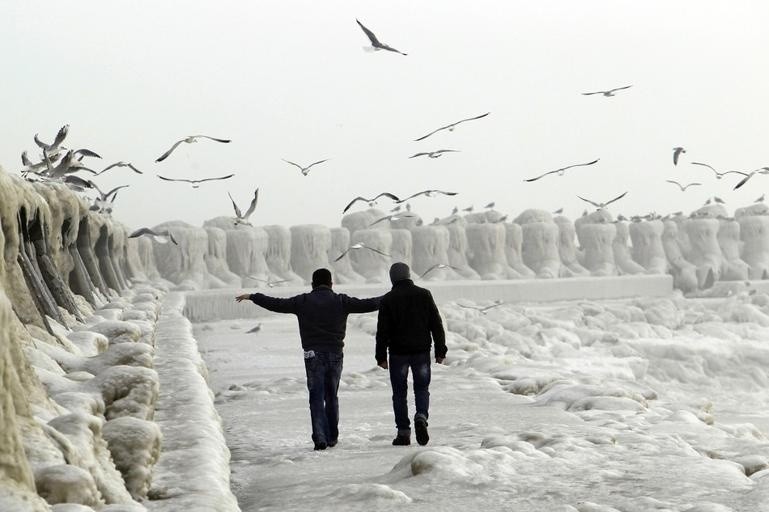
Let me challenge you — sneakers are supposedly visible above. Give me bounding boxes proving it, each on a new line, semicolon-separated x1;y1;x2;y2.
393;438;410;445
415;418;429;445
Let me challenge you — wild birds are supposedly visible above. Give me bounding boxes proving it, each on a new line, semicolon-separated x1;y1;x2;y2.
419;263;461;278
458;302;505;314
156;174;234;188
20;123;144;215
246;322;261;334
553;208;563;214
524;158;601;182
281;158;329;176
413;112;490;141
666;146;769;205
334;242;392;262
581;84;633;96
228;187;260;227
408;149;461;158
154;135;232;162
342;189;508;226
245;275;292;287
577;191;697;224
354;18;409;56
128;228;178;245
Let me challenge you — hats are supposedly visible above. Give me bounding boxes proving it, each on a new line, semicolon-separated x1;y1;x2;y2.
390;263;410;281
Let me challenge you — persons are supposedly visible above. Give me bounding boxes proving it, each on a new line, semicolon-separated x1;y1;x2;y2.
375;262;448;446
236;268;381;450
